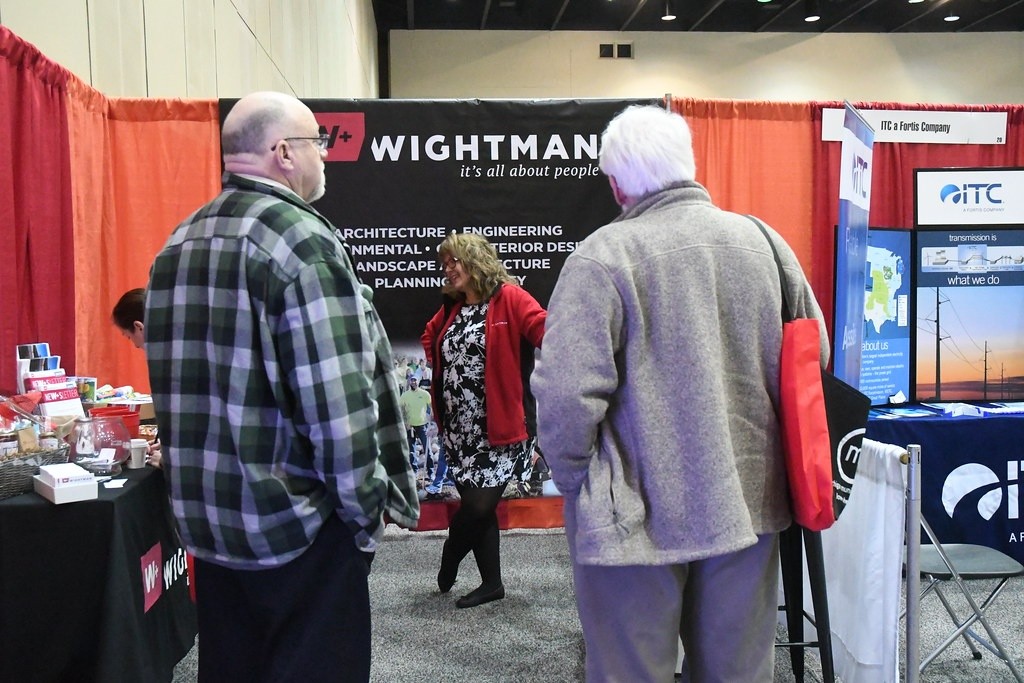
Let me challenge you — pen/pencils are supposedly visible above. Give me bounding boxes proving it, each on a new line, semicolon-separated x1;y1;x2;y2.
154;431;160;444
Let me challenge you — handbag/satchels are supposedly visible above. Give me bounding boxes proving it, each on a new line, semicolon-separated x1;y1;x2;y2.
743;212;871;530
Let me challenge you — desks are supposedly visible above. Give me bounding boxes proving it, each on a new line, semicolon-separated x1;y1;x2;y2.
0;464;162;518
866;403;1024;435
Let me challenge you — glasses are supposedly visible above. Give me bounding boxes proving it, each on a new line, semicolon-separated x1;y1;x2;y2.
271;135;328;151
438;257;460;270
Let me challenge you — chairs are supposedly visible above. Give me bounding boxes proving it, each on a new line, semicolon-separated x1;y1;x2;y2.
898;511;1024;683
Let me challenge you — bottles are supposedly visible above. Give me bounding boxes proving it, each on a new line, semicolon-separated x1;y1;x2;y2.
70;417;132;477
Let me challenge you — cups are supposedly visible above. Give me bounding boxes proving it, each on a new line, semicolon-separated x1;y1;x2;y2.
127;438;148;468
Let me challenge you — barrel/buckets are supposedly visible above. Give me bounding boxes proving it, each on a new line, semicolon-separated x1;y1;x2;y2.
96;410;140;441
89;405;131;416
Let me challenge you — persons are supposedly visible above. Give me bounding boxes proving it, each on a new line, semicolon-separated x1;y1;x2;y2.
142;91;420;683
528;102;833;683
391;353;462;503
111;288;165;470
421;231;549;610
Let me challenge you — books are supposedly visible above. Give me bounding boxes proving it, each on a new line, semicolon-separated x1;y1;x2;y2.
15;342;90;437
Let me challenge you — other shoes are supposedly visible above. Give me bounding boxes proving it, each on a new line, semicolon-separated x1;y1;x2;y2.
437;541;457;591
457;584;506;608
